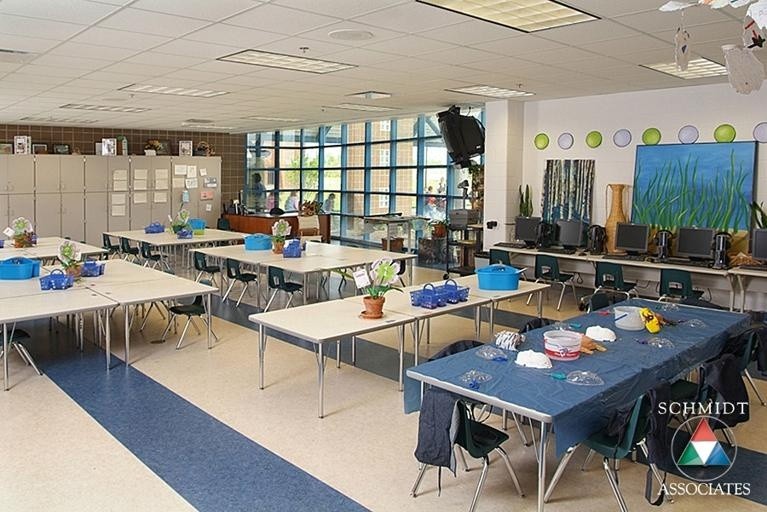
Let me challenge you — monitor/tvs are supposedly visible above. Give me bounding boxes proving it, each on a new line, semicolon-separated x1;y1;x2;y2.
514;217;541;241
437;104;484;169
675;228;715;262
613;223;649;252
751;228;767;267
552;220;582;246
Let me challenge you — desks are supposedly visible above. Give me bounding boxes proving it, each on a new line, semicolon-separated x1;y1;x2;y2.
0;237;220;392
364;216;425;253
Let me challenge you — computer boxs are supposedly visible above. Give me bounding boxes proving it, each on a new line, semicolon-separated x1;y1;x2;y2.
712;234;731;270
659;231;670;260
538;223;550;248
591;227;604;255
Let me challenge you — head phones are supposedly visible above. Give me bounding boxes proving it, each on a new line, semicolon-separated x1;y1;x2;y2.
654;229;674;247
535;220;552;237
587;224;606;241
712;232;733;253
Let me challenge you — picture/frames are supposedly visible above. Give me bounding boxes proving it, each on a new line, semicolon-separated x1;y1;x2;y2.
1;135;194;156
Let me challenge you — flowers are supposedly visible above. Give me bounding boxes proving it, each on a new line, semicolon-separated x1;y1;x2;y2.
271;218;292;243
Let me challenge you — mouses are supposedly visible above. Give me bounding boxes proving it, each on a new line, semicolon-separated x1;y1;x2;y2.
647;258;654;262
579;252;586;256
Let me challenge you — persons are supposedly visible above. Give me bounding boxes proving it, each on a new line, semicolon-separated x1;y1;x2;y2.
324;193;335;211
248;172;267;207
284;189;298;211
424;175;446;218
268;189;278;210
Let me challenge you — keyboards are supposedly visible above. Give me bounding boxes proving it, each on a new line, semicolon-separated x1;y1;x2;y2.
739;264;766;271
600;255;648;261
536;248;577;255
494;242;527;248
663;258;710;268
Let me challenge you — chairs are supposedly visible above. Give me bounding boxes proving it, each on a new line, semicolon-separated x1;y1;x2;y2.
489;244;767;313
297;215;323;243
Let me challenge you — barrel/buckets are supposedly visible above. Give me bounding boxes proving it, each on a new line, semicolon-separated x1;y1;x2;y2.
543;329;583;362
614;304;647;332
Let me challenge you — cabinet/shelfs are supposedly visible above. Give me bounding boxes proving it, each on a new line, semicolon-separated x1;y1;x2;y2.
445;224;483;276
0;154;222;247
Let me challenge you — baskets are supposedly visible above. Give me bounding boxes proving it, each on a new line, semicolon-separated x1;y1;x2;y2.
41;269;73;290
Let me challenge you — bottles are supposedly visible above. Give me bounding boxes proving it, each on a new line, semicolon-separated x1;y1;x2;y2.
121;136;128;155
